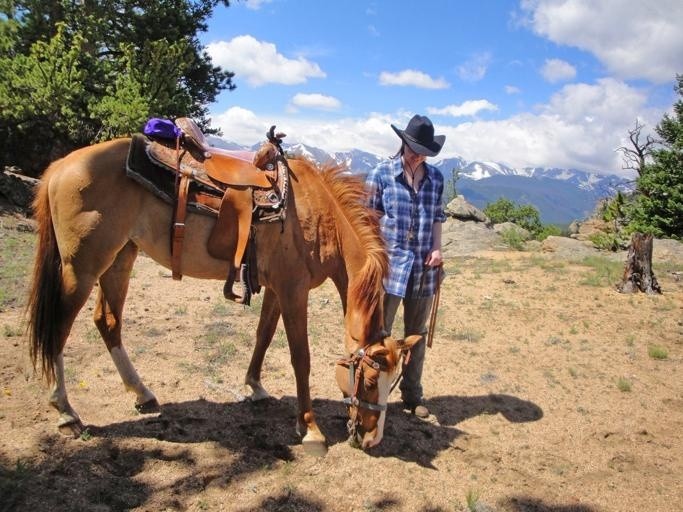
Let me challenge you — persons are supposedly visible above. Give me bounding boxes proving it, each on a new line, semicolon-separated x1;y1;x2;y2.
361;112;450;417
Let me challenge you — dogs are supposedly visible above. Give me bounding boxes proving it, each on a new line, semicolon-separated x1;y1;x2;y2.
14;134;423;458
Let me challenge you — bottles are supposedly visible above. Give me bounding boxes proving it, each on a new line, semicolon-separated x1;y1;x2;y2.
391;114;447;157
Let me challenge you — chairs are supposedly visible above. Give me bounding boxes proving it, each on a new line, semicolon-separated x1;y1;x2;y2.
401;403;429;417
